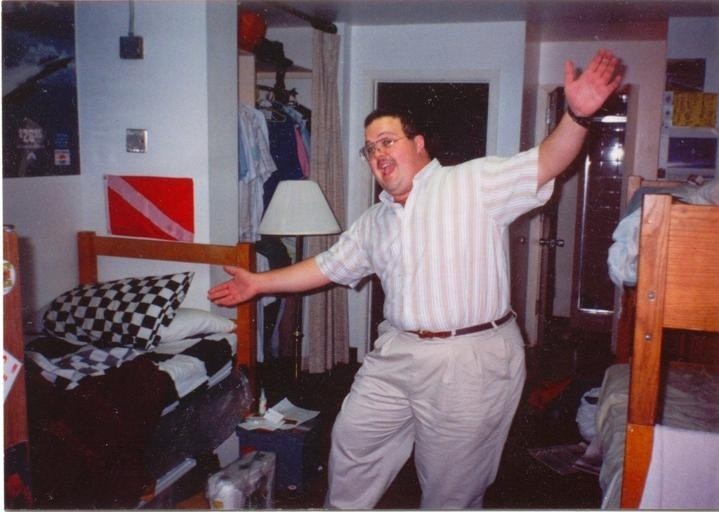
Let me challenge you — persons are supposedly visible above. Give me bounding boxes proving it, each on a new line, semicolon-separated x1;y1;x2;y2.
208;48;621;509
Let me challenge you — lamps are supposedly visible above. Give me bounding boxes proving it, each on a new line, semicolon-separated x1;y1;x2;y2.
255;178;344;408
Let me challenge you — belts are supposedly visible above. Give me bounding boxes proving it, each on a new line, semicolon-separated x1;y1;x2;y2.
403;311;513;340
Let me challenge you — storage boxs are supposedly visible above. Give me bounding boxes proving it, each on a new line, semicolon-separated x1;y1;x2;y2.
205;405;323;508
661;124;718;172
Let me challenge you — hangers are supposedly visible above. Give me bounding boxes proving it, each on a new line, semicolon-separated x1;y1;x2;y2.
256;87;288;124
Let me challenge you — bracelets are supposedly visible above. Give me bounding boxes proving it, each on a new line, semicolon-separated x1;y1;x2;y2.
568;106;592;128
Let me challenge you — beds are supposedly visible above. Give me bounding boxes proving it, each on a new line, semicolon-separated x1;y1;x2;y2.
3;230;262;508
601;174;719;507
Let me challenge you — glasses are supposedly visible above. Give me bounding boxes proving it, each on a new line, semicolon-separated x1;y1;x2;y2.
359;136;407;161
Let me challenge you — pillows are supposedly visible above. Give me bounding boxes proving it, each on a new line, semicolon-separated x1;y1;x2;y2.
29;270;237;352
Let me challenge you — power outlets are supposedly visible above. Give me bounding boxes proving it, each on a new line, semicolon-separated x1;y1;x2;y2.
126;129;147;153
